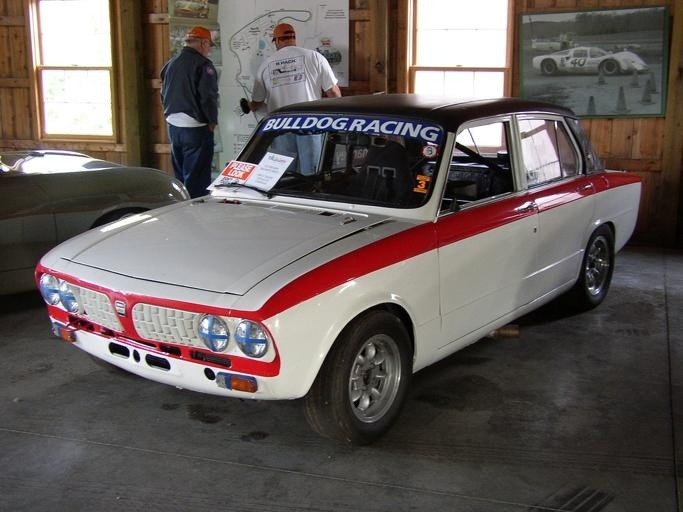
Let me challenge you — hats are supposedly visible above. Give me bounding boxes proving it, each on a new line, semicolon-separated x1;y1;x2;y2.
186;26;216;47
271;24;296;43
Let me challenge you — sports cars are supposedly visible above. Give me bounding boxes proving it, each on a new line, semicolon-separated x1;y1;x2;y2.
531;46;649;78
530;36;581;52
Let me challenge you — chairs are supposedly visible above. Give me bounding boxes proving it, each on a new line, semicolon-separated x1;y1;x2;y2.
330;137;406;208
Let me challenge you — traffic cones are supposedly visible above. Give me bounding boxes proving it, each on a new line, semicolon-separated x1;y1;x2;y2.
638;83;656;106
612;86;633;115
626;67;641;88
584;95;597;115
643;72;659;94
594;68;610;86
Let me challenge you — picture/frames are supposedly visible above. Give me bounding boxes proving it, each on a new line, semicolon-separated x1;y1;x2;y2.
509;3;669;121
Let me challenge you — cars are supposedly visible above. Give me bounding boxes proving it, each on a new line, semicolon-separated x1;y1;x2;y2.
30;92;644;445
0;146;191;301
171;1;209;18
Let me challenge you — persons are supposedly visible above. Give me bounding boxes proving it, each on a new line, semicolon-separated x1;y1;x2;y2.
159;26;218;200
249;23;341;177
559;30;568;50
